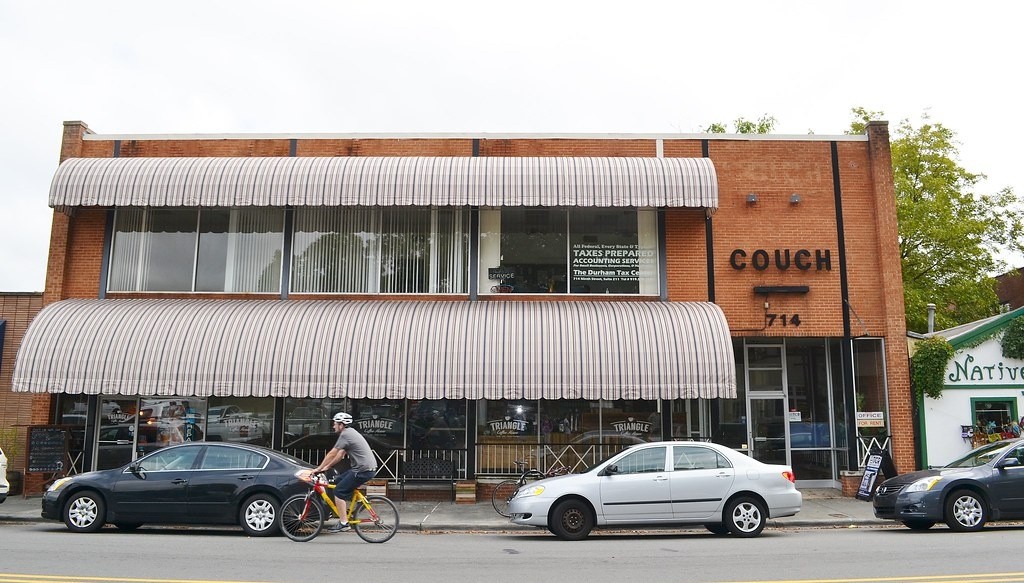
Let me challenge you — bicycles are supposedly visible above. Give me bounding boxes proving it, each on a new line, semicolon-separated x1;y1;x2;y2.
491;461;572;518
279;467;400;544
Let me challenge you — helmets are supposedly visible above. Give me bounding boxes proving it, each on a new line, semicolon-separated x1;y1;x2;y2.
333;412;353;424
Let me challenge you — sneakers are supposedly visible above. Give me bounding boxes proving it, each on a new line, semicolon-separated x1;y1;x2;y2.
327;520;351;532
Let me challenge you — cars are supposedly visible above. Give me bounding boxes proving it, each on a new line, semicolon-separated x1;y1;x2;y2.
41;445;340;538
520;430;646;473
62;396;345;453
282;432;411;477
872;439;1024;533
761;430;827;470
508;440;802;541
353;417;455;452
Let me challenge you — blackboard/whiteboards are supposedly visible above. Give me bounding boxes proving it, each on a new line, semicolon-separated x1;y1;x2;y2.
854;449;886;502
25;424;71;474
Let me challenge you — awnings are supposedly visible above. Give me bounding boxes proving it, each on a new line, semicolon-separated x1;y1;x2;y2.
47;158;721;211
8;295;738;399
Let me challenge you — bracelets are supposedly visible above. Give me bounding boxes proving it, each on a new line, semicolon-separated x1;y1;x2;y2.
315;469;321;472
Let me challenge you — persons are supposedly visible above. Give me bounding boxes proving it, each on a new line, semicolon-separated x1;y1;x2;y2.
424;409;451;454
308;412;378;532
165;399;195;441
